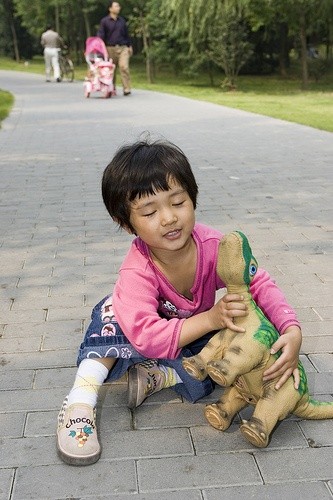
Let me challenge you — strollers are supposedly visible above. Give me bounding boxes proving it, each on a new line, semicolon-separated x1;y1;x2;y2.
82;36;116;98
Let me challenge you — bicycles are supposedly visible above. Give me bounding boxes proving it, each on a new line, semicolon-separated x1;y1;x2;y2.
57;50;75;83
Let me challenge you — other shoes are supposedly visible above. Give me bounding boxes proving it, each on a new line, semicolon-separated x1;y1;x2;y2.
56;395;102;466
46;81;50;82
123;92;130;95
128;358;166;408
57;78;59;82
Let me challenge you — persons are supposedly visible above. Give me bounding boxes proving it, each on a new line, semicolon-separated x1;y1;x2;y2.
94;0;134;97
56;130;302;466
40;24;68;82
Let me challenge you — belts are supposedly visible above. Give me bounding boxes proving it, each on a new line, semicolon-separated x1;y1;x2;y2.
111;44;128;48
